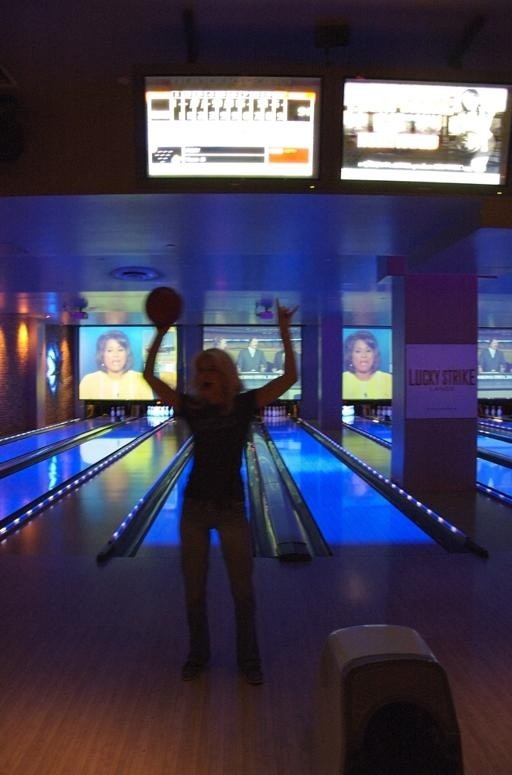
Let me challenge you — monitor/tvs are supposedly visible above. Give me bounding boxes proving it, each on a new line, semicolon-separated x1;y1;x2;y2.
141;73;324;185
337;76;512;193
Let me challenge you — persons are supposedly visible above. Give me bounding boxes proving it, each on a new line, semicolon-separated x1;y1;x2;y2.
80;330;158;401
233;333;269;374
478;336;504;373
273;338;301;380
212;335;228;356
142;293;304;685
341;330;393;402
98;431;157;505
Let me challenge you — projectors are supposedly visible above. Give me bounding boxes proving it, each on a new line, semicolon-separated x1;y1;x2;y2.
72;312;88;319
259;312;272;319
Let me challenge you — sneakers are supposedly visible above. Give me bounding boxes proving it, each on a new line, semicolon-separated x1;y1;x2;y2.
182;655;213;680
239;662;266;685
